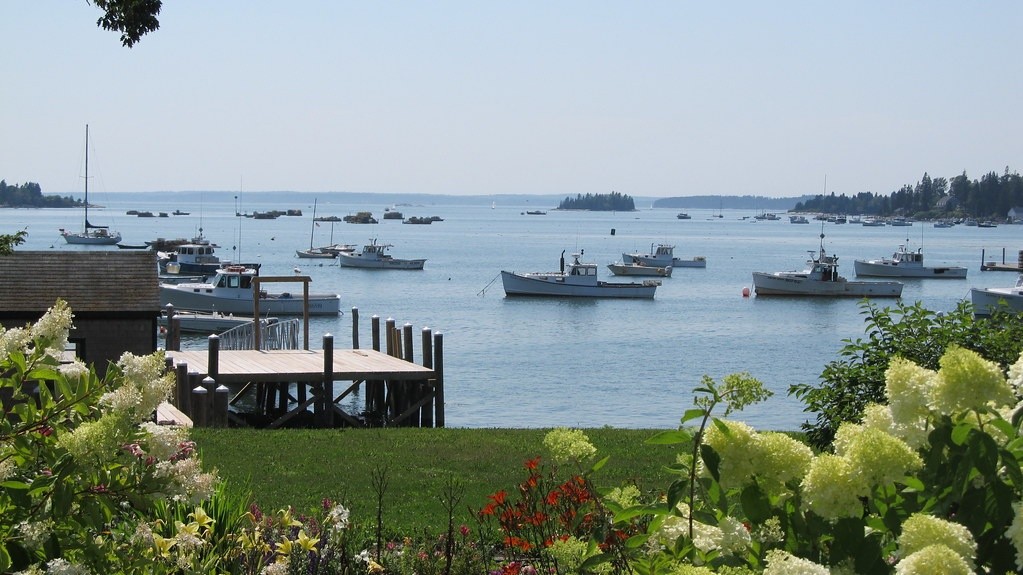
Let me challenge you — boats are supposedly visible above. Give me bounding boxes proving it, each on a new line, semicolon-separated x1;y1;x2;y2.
752;173;905;298
172;210;191;215
934;217;997;227
854;221;968;279
157;304;279;335
737;209;912;227
621;232;707;268
606;263;672;277
527;210;547;215
126;209;169;217
501;231;662;299
676;212;692;219
234;195;302;219
159;175;341;317
314;207;444;224
296;198;428;269
296;198;333;258
116;243;148;249
970;275;1023;318
145;192;261;285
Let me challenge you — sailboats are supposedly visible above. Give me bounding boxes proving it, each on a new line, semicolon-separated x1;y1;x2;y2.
59;124;121;244
